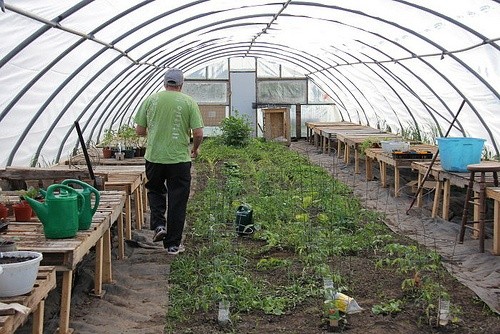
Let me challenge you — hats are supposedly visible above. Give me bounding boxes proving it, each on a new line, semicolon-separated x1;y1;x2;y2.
164;68;184;86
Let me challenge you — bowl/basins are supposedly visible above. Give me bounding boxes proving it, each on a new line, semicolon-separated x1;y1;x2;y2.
0;251;42;295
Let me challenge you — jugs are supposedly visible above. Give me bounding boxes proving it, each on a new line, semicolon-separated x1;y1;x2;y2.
24;180;78;239
58;180;100;229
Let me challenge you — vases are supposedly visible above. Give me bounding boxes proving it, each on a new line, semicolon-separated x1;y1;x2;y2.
0;251;43;297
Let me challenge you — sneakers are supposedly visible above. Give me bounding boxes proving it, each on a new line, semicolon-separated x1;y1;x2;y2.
168;244;185;254
153;226;167;242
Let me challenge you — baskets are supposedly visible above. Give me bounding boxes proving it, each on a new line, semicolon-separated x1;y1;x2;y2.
380;140;410;154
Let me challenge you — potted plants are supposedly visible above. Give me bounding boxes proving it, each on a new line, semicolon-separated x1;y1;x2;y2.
0;124;145;221
0;236;20;252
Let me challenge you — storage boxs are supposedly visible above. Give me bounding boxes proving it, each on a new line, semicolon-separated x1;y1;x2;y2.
433;137;487;172
392;150;433;160
381;141;410;155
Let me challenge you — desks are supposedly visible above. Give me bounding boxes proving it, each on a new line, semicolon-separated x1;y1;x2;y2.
305;121;500;255
0;135;149;334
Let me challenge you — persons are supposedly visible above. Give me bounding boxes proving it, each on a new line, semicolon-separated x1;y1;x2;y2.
133;69;204;255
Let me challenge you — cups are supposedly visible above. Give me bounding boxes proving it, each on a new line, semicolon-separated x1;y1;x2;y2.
438;301;451;325
218;300;230;324
323;272;337;289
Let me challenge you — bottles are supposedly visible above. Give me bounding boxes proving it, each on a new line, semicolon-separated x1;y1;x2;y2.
334;290;367;314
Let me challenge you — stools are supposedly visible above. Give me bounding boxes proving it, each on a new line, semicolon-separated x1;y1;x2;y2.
458;163;500;253
